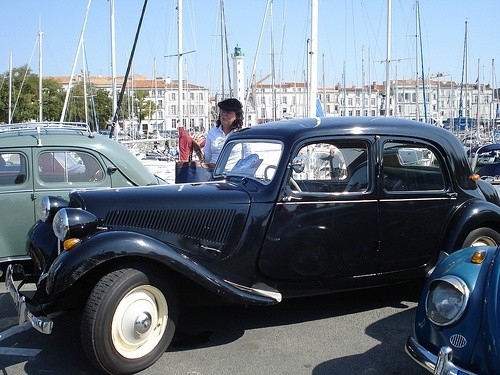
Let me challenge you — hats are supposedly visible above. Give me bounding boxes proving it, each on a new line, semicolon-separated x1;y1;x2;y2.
218;98;241;112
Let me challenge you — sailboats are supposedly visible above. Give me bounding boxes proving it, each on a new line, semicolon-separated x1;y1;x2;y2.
0;0;500;184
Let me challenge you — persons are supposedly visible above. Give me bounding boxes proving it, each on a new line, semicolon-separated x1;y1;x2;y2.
152;140;170;153
203;99;255;173
298;150;344;180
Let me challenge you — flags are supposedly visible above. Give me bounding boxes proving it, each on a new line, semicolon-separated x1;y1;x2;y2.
315;94;324;118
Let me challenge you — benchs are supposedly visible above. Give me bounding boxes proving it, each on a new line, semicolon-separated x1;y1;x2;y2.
383;166;445;191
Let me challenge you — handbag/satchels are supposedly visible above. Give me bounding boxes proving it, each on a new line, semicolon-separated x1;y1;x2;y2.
175;141;216;184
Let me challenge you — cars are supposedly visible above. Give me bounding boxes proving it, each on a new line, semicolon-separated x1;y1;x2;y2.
0;121;171;283
5;117;500;375
